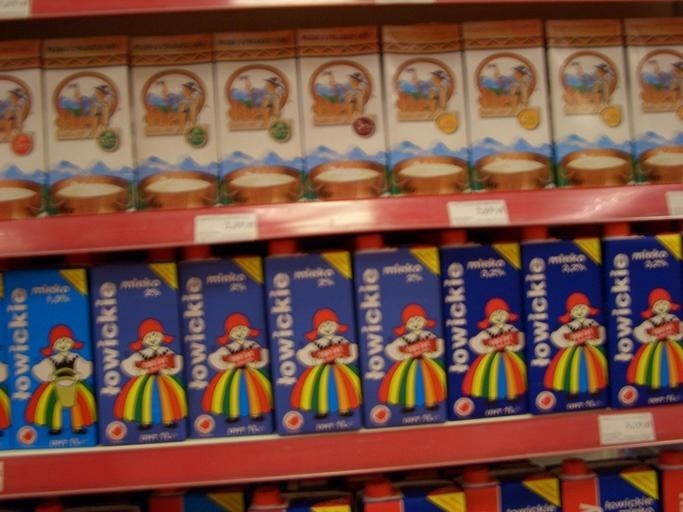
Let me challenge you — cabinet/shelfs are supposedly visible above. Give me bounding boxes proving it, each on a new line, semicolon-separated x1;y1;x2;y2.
1;2;682;512
